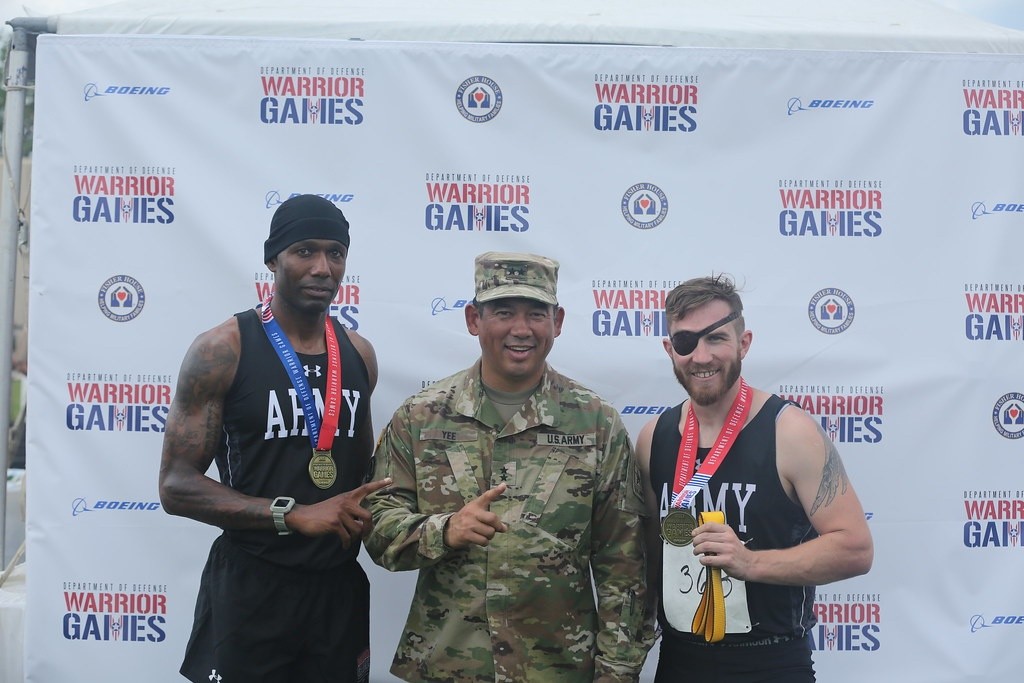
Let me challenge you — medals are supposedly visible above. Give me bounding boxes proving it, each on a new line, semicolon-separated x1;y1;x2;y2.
308;448;337;489
661;509;698;547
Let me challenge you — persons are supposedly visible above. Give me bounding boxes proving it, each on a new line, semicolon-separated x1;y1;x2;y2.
635;278;874;683
362;252;655;683
157;195;380;683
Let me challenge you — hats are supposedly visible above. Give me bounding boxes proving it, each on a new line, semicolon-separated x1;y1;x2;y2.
474;251;559;307
264;195;350;261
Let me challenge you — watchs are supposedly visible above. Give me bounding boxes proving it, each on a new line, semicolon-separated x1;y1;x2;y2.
269;496;298;537
